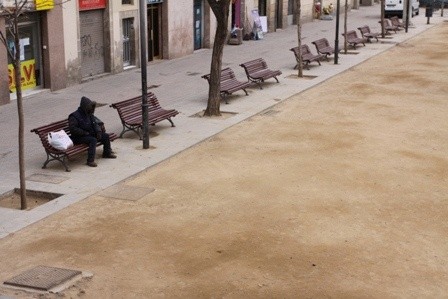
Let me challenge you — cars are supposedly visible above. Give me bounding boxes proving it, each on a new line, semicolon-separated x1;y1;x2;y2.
384;0;421;18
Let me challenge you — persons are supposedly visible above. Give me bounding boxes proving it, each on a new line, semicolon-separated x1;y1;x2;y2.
68;96;116;167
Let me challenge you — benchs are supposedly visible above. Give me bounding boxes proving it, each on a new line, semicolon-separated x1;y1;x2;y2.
30;119;117;173
109;92;180;141
341;29;367;50
290;43;323;71
239;57;283;90
389;16;404;30
378;19;398;34
357;25;382;44
311;38;341;63
201;66;253;105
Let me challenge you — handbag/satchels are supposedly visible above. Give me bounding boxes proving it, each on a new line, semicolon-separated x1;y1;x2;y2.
48;130;73;151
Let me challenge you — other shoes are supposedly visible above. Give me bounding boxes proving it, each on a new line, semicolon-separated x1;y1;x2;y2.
87;162;97;167
102;154;117;158
